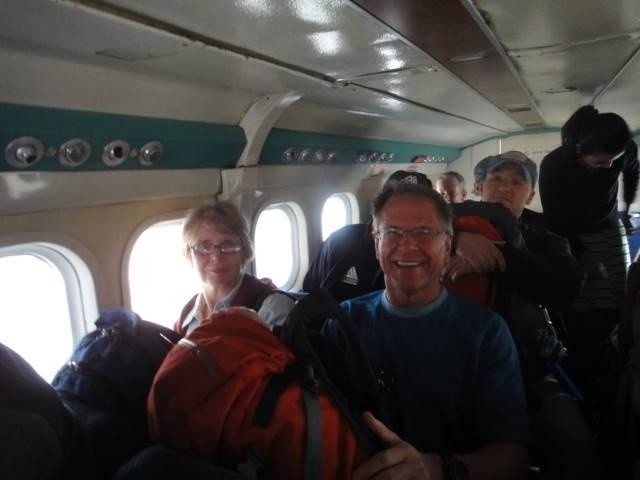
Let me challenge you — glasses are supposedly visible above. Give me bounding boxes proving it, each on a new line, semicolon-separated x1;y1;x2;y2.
369;227;447;241
185;240;246;255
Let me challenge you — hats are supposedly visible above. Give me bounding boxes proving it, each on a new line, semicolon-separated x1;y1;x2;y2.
486;149;538;191
